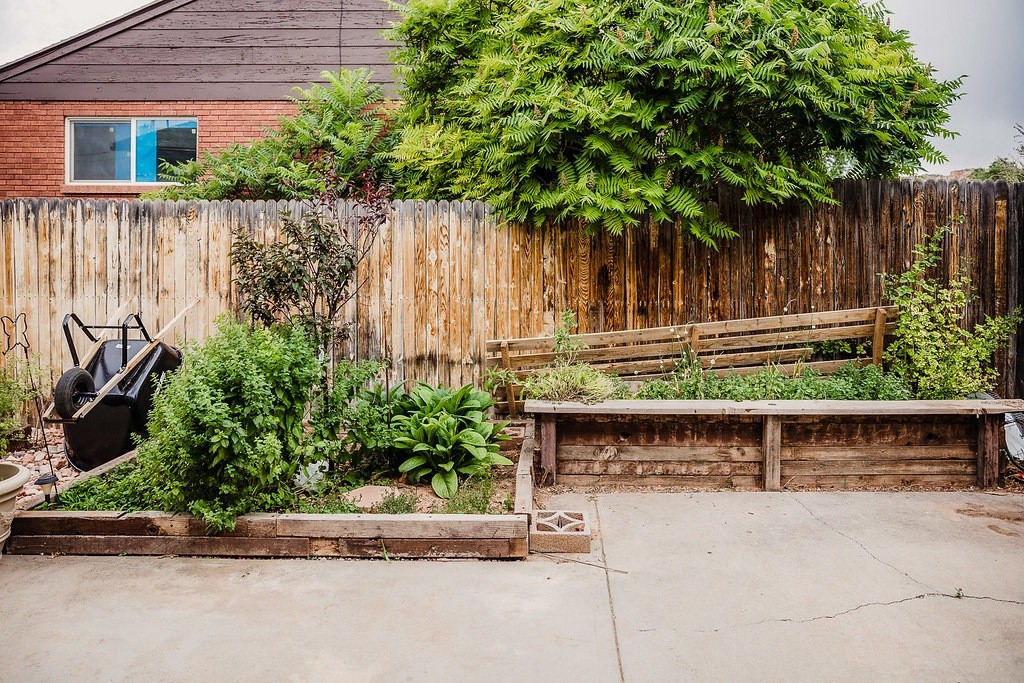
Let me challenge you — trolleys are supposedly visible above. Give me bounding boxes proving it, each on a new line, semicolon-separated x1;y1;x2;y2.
42;313;184;470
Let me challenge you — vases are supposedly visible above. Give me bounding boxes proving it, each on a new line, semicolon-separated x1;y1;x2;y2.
0;460;32;557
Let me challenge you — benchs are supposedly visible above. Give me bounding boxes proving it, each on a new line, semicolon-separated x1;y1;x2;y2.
523;399;1024;489
